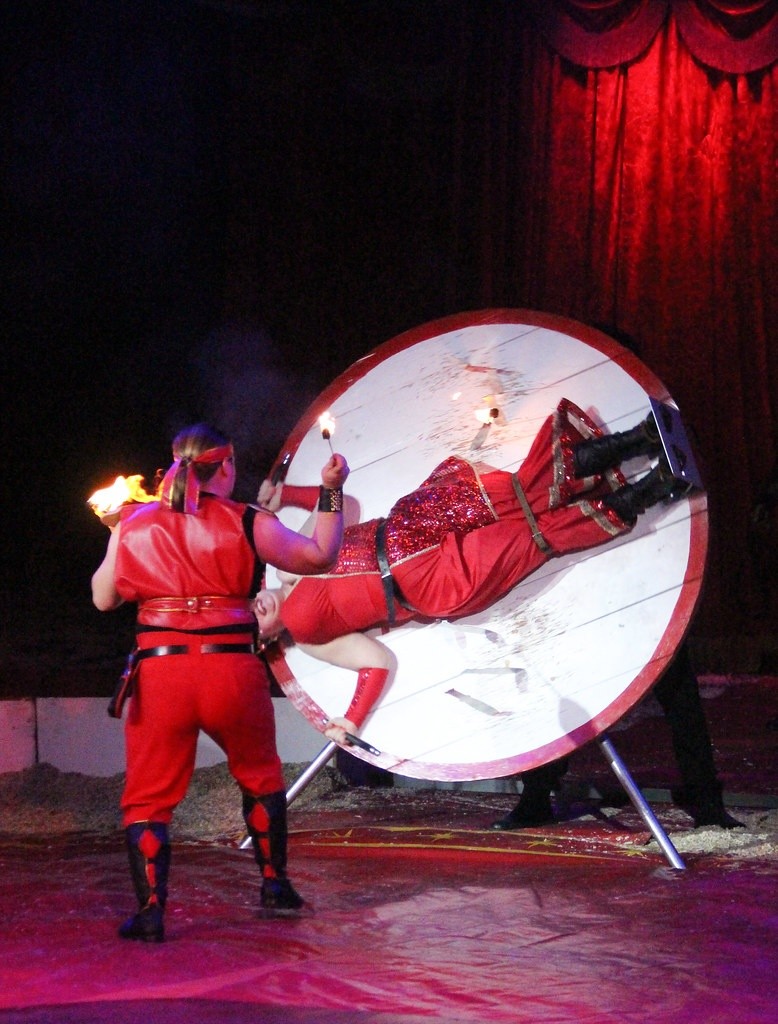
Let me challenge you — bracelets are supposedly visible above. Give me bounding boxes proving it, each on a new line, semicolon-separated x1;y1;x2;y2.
317;485;343;513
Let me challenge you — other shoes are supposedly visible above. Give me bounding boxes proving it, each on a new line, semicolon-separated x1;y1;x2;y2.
491;791;554;829
261;878;303;908
692;807;745;830
119;909;163;942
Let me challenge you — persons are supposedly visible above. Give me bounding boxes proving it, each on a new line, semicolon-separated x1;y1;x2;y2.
486;319;748;833
90;422;350;942
258;397;705;673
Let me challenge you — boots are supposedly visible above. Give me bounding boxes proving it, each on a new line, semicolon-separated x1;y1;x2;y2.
574;411;661;478
605;449;687;525
